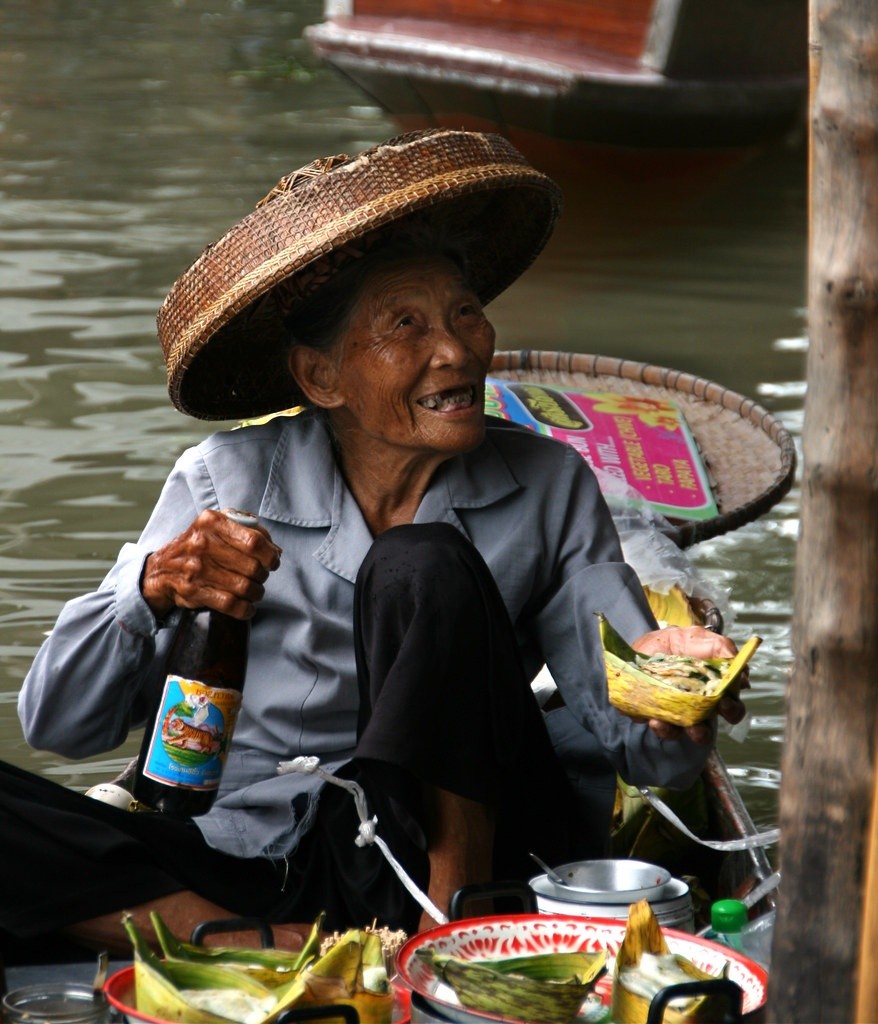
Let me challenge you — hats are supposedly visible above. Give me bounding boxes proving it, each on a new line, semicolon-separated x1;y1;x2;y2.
157;128;557;420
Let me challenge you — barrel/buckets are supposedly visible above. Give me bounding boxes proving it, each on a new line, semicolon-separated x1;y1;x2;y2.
529;873;695;934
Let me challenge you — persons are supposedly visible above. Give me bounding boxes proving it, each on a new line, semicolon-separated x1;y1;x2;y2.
0;129;750;964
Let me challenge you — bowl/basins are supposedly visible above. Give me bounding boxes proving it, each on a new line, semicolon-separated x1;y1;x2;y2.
548;861;671;902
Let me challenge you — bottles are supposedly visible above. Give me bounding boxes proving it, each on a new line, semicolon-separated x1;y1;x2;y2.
133;509;259;817
700;900;748;955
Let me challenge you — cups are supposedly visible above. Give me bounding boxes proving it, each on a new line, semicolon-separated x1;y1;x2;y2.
2;982;110;1024
